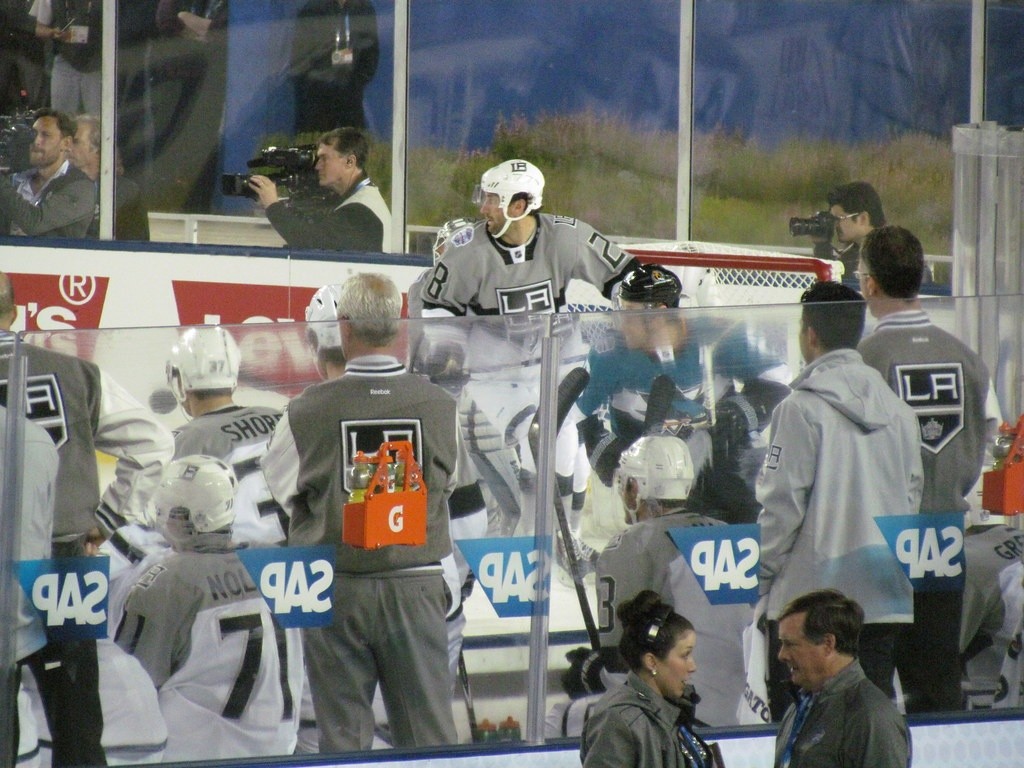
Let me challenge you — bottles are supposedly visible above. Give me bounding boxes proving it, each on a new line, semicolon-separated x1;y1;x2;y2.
372;449;394;495
395;444;421;492
474;716;520;744
994;421;1014;471
348;450;371;504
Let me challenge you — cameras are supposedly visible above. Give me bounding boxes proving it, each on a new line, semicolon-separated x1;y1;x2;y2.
789;212;835;238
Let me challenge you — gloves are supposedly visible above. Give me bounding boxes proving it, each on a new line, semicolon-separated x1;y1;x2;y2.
576;414;626;488
713;378;791;446
559;647;629;697
424;343;470;389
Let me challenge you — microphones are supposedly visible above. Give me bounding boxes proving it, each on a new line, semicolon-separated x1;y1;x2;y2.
247;157;265;167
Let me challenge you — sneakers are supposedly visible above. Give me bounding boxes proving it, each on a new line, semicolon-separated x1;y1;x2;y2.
556;533;596;588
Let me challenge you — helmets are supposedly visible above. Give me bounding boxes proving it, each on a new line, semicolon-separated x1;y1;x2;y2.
616;433;694;498
156;455;241;532
480;158;546;210
168;327;243;403
617;263;683;321
305;284;347;347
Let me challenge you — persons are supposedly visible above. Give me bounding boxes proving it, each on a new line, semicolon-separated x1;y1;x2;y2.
0;0;228;243
0;227;1024;768
421;160;640;589
773;589;912;768
580;590;726;768
812;182;886;291
249;127;394;253
287;0;379;133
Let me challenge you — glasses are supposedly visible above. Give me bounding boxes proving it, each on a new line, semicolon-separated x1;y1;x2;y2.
853;269;873;281
834;211;861;228
